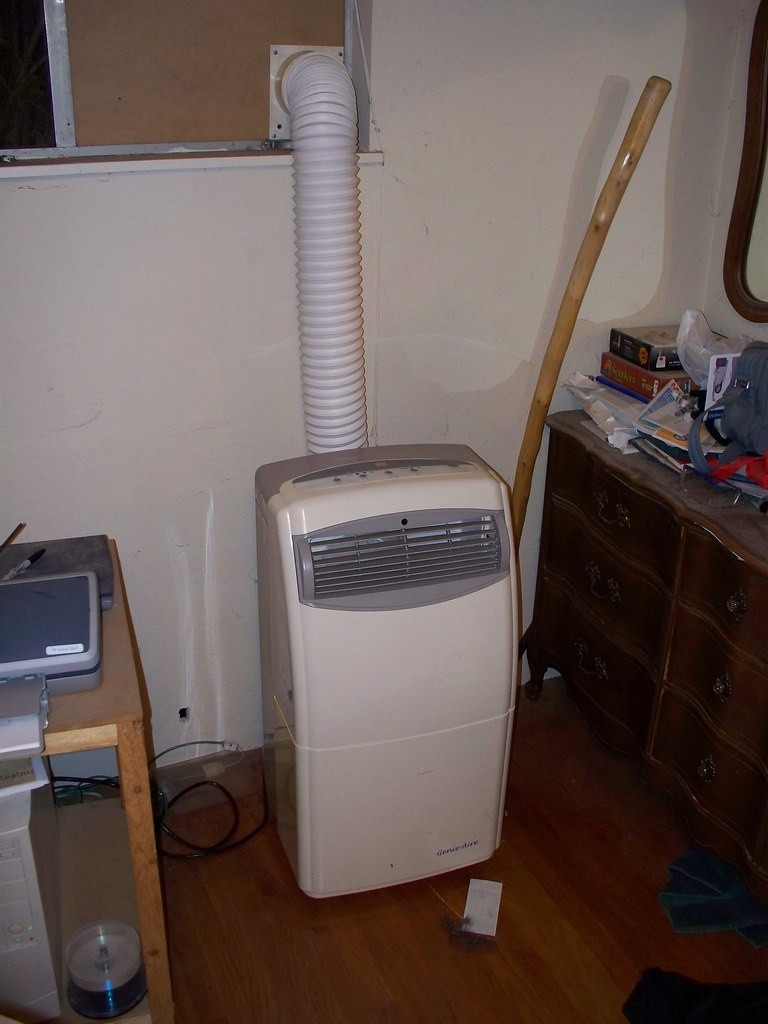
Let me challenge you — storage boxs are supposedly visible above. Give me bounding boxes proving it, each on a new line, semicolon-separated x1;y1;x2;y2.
600;327;728;399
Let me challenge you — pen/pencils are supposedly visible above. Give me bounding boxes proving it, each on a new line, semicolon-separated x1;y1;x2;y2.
2;548;46;581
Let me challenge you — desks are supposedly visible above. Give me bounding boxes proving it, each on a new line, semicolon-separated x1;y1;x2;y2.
0;539;176;1024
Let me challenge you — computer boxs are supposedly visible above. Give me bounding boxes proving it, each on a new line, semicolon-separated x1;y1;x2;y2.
0;755;63;1024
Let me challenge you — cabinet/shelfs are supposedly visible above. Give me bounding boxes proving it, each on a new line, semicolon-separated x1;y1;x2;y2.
523;410;768;911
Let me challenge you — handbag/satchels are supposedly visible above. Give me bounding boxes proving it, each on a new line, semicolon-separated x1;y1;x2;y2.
687;340;768;475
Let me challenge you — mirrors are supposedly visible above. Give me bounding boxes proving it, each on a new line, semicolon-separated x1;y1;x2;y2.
723;0;768;326
